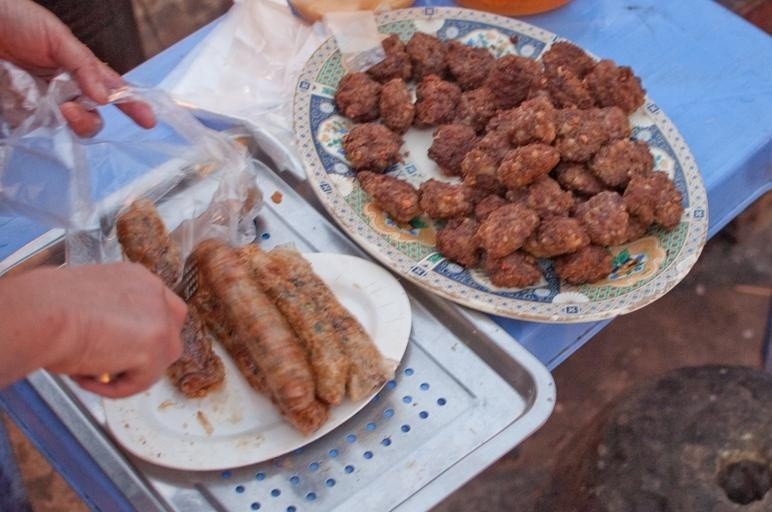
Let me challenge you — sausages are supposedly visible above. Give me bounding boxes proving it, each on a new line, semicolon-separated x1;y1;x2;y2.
118;202;386;437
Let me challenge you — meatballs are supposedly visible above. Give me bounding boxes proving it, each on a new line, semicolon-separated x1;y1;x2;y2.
337;32;682;285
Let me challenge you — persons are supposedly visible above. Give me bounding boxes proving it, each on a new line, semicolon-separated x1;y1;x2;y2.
0;1;187;399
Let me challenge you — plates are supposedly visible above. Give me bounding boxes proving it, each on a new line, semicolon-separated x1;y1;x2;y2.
291;6;710;325
92;252;412;472
